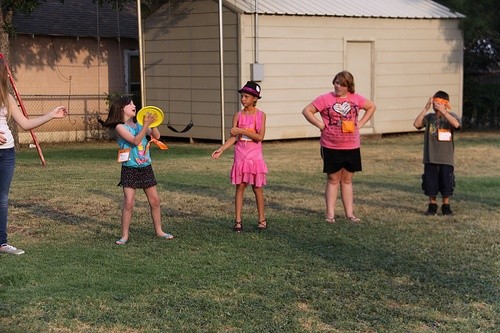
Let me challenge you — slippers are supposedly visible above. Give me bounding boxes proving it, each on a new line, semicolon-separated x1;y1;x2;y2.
324;217;335;223
348;217;360;222
115;239;126;245
159;233;174;239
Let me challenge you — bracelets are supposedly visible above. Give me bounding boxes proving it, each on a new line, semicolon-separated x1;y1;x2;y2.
444;112;448;115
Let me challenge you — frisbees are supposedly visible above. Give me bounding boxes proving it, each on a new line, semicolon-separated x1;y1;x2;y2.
136;105;164;128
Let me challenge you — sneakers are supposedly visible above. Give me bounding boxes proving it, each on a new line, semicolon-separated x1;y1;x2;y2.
0;243;25;255
441;203;453;216
424;202;438;216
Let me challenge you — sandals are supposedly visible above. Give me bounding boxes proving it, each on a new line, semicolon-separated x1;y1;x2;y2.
235;218;242;234
258;218;267;229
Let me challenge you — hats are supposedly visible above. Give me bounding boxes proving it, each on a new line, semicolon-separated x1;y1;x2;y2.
237;80;262;100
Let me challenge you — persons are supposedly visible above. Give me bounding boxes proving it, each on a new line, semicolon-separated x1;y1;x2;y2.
103;95;173;245
0;53;67;254
413;91;460;215
302;71;376;222
212;81;267;231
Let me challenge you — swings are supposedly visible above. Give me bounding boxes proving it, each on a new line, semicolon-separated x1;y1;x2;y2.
166;0;195;133
96;0;123;129
